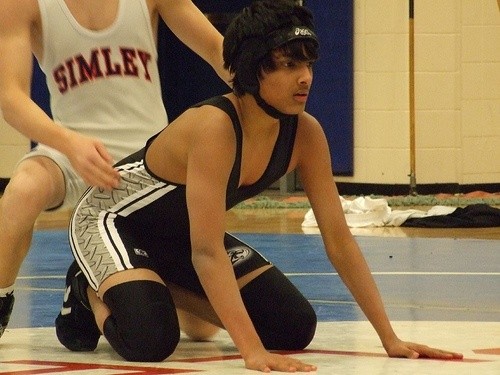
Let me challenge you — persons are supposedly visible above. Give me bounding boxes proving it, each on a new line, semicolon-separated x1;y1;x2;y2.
0;0;236;339
57;0;463;373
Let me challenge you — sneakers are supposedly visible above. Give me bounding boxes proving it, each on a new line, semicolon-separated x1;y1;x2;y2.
55;259;101;352
0;290;15;339
